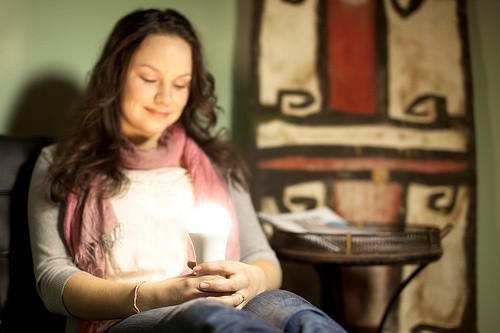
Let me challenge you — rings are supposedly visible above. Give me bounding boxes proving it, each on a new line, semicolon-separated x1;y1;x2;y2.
231;293;244;302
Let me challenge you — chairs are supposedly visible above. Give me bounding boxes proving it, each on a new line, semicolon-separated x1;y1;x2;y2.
0;135;67;333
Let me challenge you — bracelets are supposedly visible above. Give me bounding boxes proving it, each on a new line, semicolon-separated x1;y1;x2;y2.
132;280;145;314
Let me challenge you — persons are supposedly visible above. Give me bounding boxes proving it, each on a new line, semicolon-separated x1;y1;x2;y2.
28;8;348;333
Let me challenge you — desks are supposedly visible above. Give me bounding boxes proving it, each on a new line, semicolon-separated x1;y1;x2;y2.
268;222;443;333
